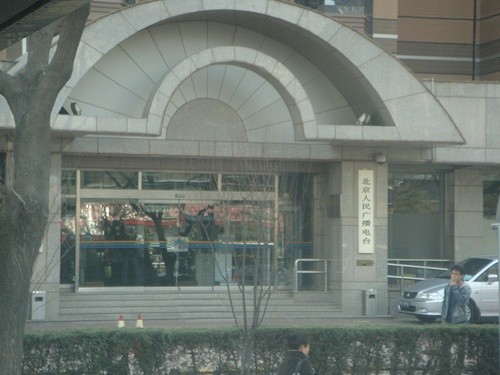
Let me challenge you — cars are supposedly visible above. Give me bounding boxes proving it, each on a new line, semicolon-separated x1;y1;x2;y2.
398;256;499;326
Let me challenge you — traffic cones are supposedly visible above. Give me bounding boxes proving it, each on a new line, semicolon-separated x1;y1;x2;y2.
116;315;128;356
132;315;147;356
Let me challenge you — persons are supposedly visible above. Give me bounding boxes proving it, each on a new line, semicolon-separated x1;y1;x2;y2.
437;265;472;366
